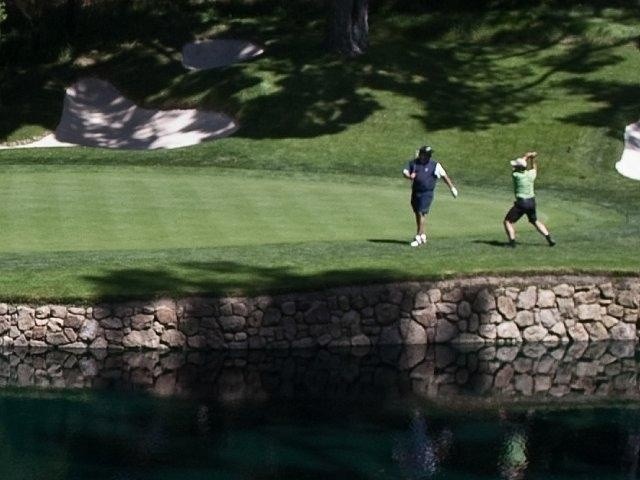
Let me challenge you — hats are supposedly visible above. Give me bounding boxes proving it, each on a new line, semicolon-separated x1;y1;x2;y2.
419;145;433;154
510;158;527;168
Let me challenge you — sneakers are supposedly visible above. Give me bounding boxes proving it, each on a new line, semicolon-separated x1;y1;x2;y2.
410;234;426;247
550;241;556;245
504;243;515;248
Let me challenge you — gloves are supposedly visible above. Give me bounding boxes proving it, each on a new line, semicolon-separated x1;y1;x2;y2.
451;187;457;197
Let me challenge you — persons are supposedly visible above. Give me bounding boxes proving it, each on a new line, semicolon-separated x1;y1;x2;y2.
503;152;556;248
402;146;458;247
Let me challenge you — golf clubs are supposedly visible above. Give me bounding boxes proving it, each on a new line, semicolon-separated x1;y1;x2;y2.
412;149;420;173
